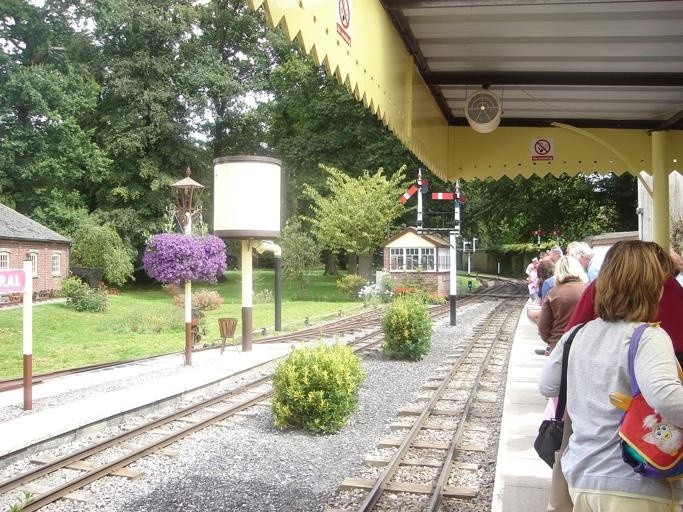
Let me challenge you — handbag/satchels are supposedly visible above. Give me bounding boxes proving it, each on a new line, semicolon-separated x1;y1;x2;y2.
546;450;574;512
534;417;564;468
608;392;683;479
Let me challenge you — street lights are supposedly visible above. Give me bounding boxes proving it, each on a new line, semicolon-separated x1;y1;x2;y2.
170;166;206;367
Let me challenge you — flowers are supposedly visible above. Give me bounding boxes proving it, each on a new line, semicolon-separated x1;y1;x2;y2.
144;233;227;284
